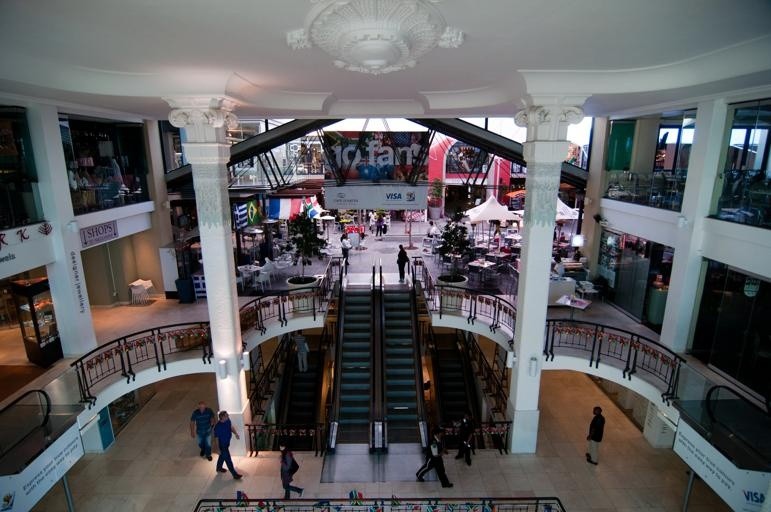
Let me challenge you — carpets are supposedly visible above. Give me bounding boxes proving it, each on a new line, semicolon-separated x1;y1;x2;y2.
1;365;56;403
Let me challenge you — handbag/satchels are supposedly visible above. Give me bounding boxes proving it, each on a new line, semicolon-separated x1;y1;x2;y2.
286;459;300;477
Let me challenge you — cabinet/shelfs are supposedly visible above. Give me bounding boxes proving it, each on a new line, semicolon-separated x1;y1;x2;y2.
13;276;64;369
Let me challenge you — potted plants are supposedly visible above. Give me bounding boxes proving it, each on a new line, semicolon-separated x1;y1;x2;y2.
286;211;330;312
428;177;472;313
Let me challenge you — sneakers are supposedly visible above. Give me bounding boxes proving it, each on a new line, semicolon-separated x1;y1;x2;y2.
200;449;242;480
416;454;472;488
586;452;598;465
298;488;306;497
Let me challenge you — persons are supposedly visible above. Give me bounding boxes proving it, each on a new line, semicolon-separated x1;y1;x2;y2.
341;233;351;265
397;245;409;282
428;221;441;238
279;443;304;499
453;412;475;466
214;411;243;480
552;256;565;276
369;211;385;237
190;400;216;461
295;330;310;372
586;407;605;465
416;429;453;488
258;243;266;266
